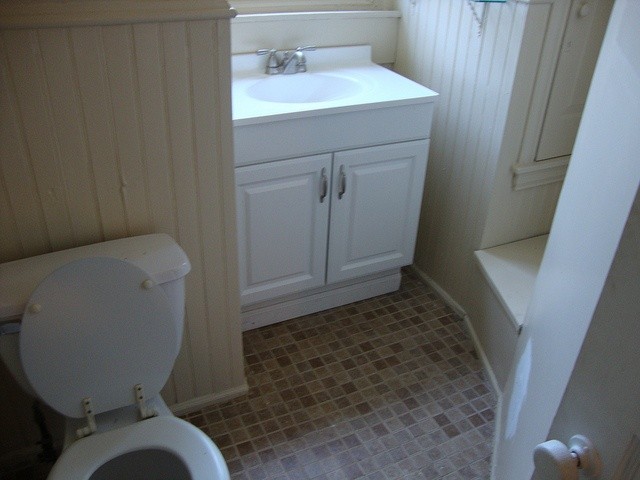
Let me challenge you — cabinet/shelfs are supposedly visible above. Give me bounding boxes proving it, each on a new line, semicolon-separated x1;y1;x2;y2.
234;138;431;334
535;1;616;162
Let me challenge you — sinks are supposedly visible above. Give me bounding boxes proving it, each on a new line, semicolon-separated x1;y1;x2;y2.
244;75;364;104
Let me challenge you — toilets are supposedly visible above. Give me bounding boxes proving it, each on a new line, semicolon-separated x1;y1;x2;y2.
0;232;230;479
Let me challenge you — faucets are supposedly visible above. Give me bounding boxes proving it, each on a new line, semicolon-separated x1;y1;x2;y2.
281;49;307;75
